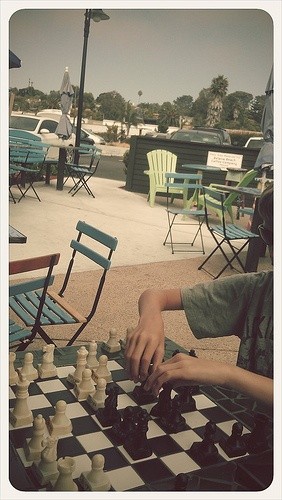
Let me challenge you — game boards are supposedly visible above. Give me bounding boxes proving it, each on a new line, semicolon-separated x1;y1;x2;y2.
8;356;268;492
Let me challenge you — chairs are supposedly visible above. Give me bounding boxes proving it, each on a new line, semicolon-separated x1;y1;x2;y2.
9;130;101;203
144;150;183;208
9;220;118;347
9;252;61;352
162;172;211;254
198;186;261;280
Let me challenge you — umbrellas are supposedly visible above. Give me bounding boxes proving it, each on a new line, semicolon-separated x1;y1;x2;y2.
9;50;21;69
55;72;74;142
254;65;274;193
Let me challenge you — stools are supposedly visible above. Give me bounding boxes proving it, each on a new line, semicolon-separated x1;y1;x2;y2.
222;168;248;219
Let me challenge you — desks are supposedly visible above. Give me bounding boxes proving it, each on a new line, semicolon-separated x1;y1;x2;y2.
45;142;81;190
182;163;221;209
10;334;273;492
214;185;267;273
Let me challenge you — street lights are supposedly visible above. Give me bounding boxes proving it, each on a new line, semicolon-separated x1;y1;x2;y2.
73;9;111;167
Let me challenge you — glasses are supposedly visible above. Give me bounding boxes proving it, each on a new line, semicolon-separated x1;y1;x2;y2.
257;222;274;247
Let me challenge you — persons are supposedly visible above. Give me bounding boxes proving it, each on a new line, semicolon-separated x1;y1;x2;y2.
125;185;273;409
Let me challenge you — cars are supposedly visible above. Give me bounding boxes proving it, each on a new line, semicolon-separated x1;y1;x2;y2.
192;125;232;145
243;137;265;149
37;109;107;146
9;114;77;167
169;130;224;146
